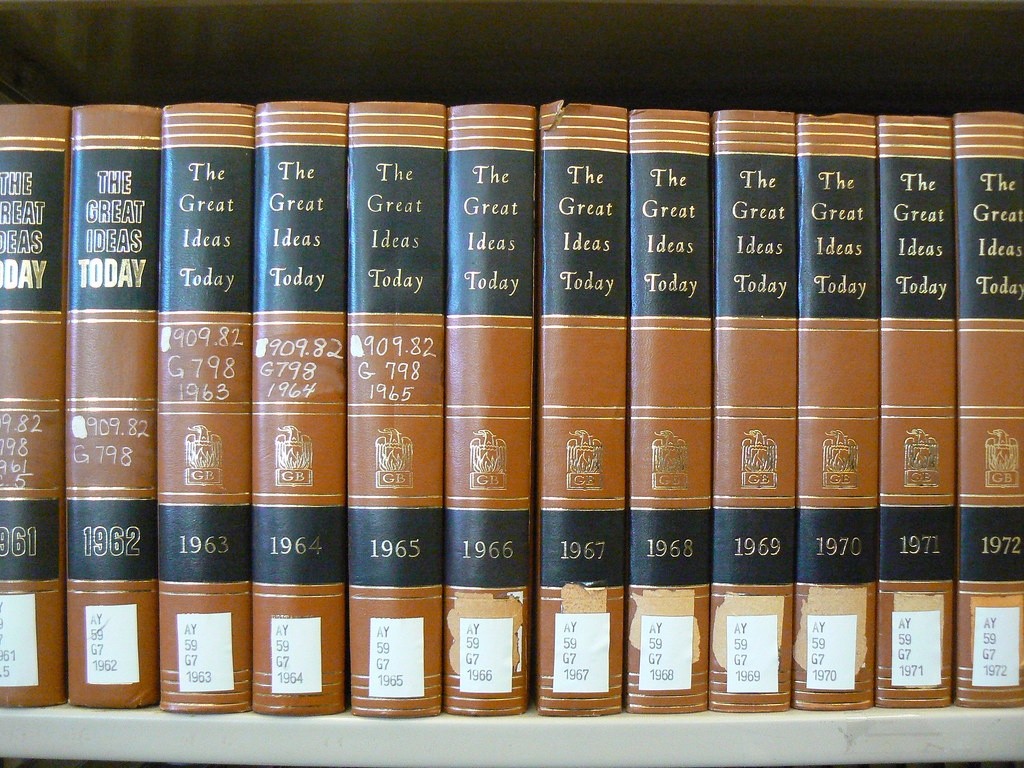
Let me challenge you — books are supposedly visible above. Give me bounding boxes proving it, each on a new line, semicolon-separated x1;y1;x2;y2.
707;109;794;711
952;110;1024;709
876;114;956;709
444;102;533;715
626;108;711;715
156;101;253;717
252;99;346;718
0;101;71;710
67;104;159;710
794;112;875;709
534;100;630;718
345;100;450;718
